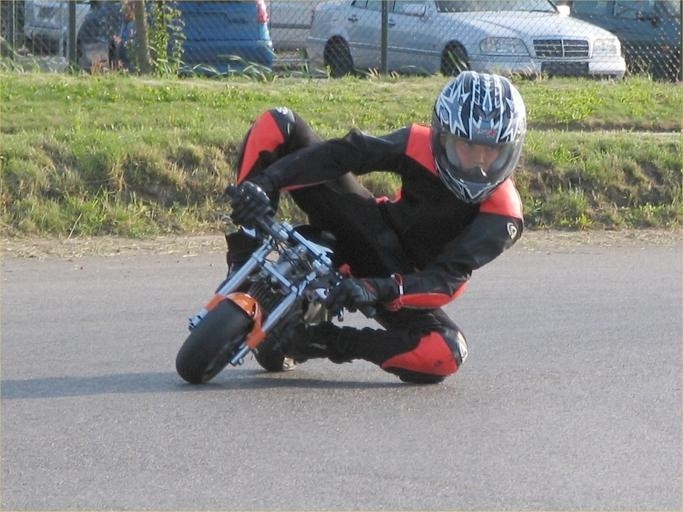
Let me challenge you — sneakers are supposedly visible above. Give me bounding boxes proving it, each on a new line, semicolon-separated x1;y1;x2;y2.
281;320;341;371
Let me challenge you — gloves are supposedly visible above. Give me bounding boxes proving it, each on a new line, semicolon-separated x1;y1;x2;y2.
229;180;272;226
323;276;400;316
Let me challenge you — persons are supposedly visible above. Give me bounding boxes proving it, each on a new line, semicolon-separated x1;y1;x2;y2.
224;70;525;387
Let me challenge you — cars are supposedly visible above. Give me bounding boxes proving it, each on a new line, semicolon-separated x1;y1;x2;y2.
22;1;313;77
570;0;683;84
304;0;628;82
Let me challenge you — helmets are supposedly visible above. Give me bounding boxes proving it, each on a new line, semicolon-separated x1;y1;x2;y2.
429;71;527;205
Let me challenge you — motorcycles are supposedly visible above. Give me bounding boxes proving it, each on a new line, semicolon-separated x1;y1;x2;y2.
173;180;380;385
